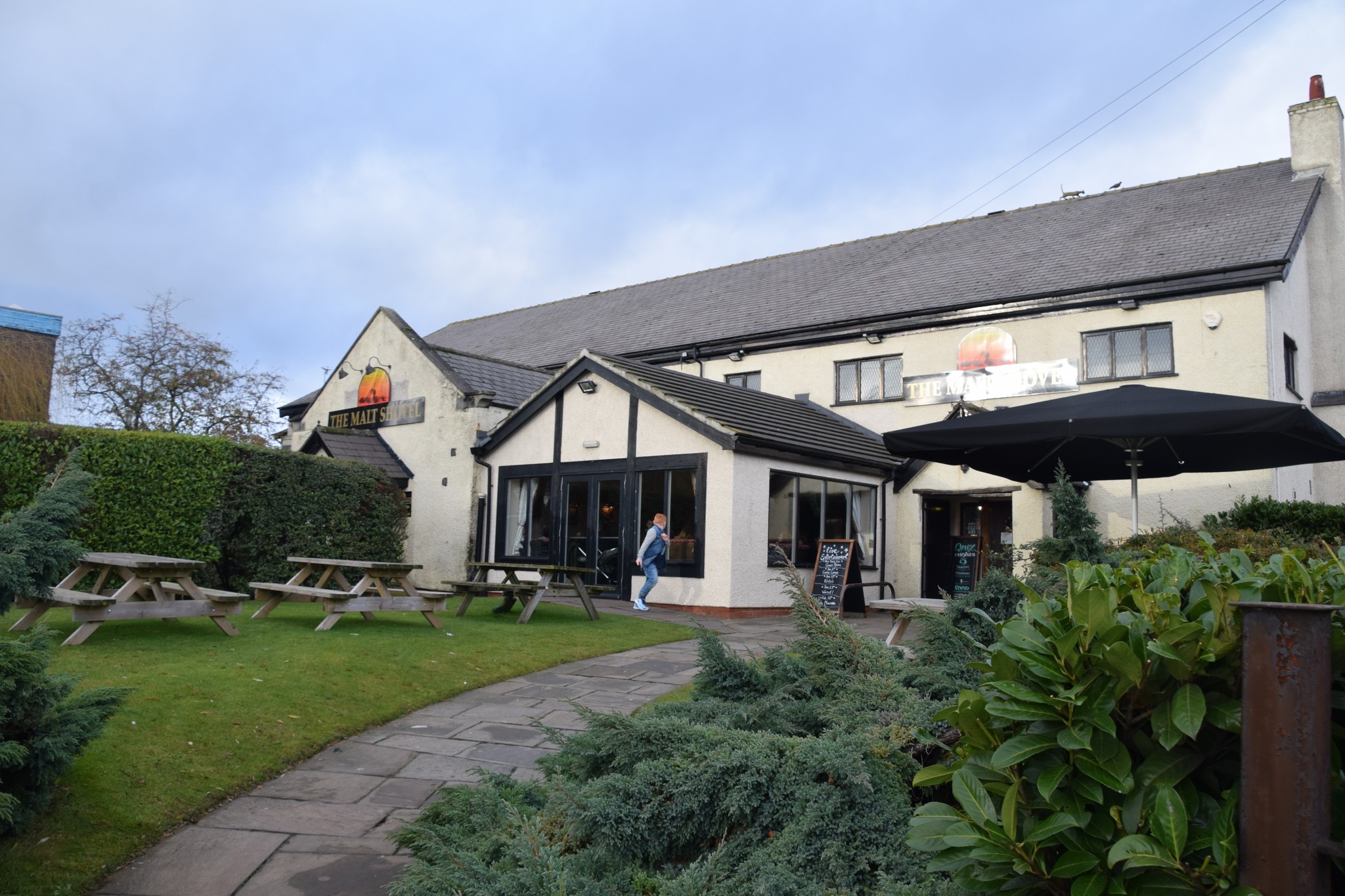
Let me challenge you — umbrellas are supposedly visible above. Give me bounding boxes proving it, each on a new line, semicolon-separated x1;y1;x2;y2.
881;384;1345;535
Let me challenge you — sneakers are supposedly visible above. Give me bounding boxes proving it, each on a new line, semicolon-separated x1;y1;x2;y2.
633;602;642;610
634;596;649;611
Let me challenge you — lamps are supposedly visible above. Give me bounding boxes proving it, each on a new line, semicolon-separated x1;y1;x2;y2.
366;357;391;375
577;380;597;393
1118;298;1139;310
865;333;882;344
726;352;743;362
338;362;363;379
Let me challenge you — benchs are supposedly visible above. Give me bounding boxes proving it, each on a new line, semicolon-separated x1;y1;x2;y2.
440;580;546;590
520;580;617;591
144;581;250;602
30;583;116;606
246;582;357;599
350;585;452;598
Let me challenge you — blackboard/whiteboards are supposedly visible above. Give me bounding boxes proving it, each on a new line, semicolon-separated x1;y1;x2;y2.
944;534;981;600
808;539;855;614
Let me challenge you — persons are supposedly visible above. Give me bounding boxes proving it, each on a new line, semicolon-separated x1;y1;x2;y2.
673;526;693;540
633;514;670;610
640;519;653;539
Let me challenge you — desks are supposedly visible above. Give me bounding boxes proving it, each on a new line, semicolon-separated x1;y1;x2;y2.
250;556;454;631
870;598;950;646
454;562;600;626
6;552;250;647
